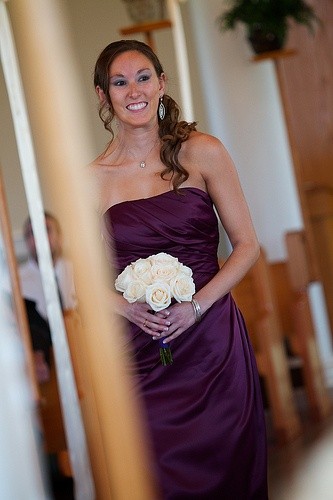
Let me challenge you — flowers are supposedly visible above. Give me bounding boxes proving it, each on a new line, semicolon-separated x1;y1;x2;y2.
114;252;196;368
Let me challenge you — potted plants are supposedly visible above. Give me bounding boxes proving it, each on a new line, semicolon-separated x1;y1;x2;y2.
215;0;316;55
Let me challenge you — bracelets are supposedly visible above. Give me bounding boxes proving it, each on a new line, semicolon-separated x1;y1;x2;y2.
191;295;201;323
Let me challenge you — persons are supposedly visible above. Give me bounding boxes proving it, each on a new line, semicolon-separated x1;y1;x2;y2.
85;40;268;500
0;211;73;383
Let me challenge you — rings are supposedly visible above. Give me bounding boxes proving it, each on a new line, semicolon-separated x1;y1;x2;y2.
143;319;147;327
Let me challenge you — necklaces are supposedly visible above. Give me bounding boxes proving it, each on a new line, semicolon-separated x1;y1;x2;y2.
117;133;159;168
